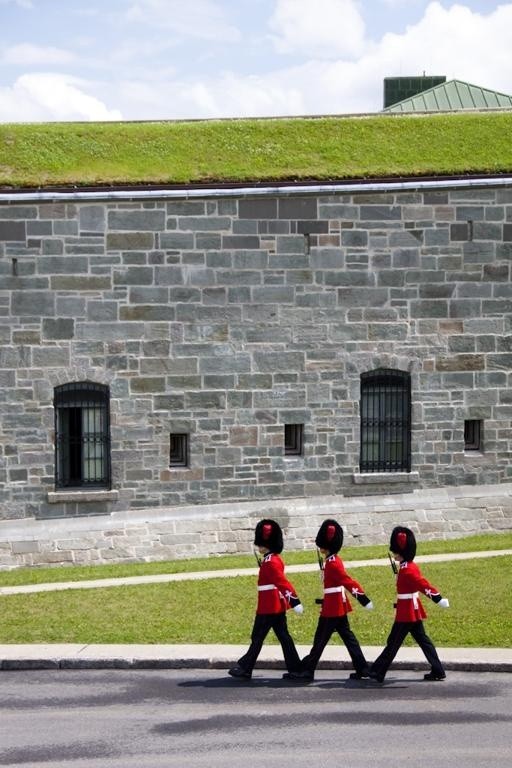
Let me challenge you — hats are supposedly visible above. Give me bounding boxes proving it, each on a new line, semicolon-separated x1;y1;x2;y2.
389;525;417;561
254;519;284;555
315;518;344;553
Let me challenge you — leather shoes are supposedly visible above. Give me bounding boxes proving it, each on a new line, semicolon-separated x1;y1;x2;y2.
281;671;316;682
228;668;252;681
349;670;387;684
421;672;447;681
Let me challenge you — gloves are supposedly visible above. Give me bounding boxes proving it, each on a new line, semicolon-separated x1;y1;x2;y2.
438;596;450;608
365;601;374;611
294;604;304;614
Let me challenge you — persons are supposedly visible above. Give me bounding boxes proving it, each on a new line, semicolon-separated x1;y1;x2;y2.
364;546;451;684
282;538;375;679
226;540;305;683
